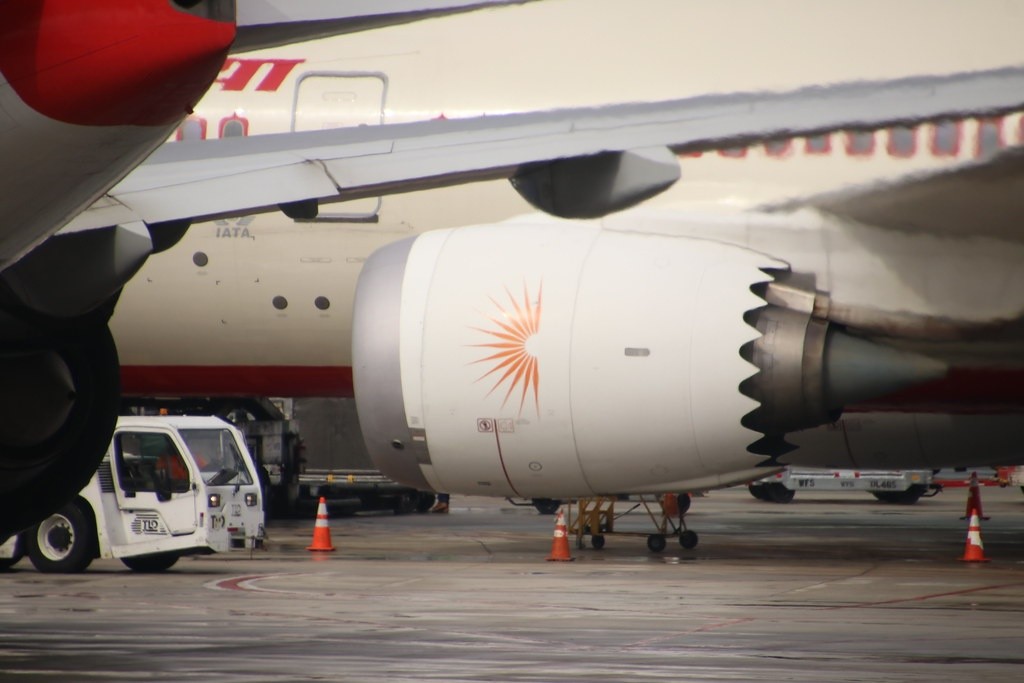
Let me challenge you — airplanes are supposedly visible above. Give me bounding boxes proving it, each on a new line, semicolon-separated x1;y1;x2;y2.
0;1;1024;537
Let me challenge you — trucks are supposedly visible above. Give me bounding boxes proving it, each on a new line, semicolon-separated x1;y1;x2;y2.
1;412;267;582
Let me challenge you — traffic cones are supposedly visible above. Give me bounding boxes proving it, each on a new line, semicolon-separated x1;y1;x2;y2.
545;506;575;561
956;506;989;564
306;497;335;551
962;470;988;522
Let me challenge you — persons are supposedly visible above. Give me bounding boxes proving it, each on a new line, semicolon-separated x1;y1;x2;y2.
148;456;176;488
